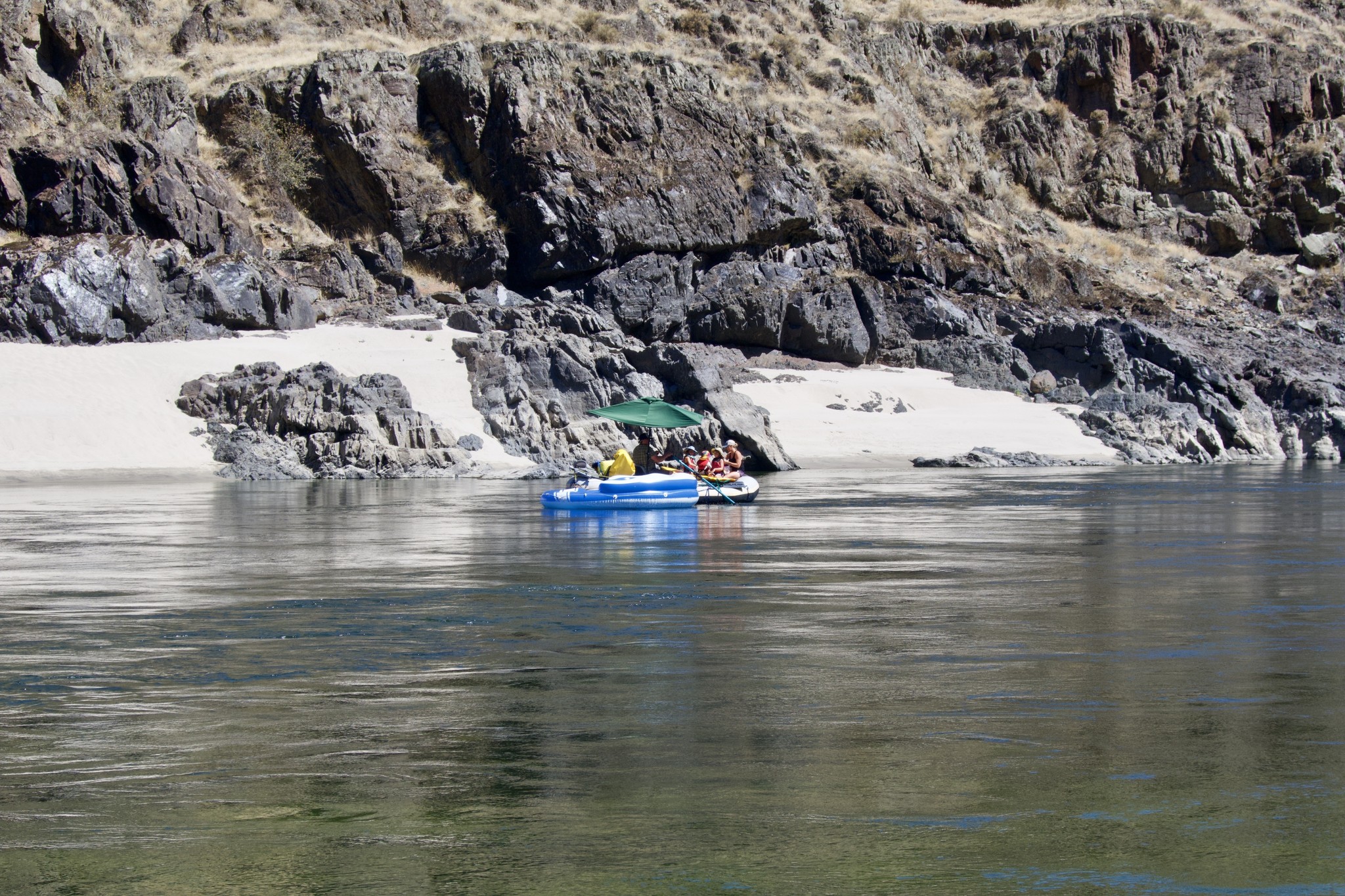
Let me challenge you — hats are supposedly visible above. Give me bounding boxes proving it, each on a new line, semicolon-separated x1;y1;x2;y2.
723;440;736;449
683;446;699;456
639;433;653;440
711;447;725;458
700;450;712;458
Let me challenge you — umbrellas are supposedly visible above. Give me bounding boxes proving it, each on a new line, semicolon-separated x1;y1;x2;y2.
585;395;713;475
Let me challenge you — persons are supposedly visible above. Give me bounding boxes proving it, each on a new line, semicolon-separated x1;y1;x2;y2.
697;451;712;476
683;446;698;474
633;433;673;475
723;440;745;479
708;447;725;478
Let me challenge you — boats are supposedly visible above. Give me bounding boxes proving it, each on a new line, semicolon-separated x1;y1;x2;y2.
540;472;700;509
565;460;760;503
540;506;698;537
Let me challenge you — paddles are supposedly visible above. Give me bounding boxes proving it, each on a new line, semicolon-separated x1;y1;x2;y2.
671;455;740;506
